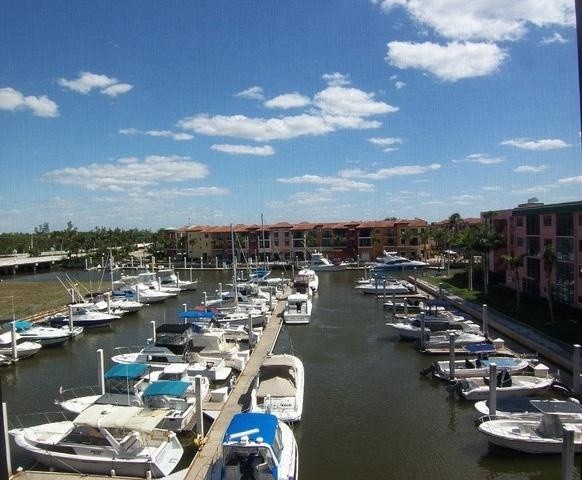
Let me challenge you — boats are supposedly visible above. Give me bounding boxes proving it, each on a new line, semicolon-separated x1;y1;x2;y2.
369;251;430;272
299;253;348;271
